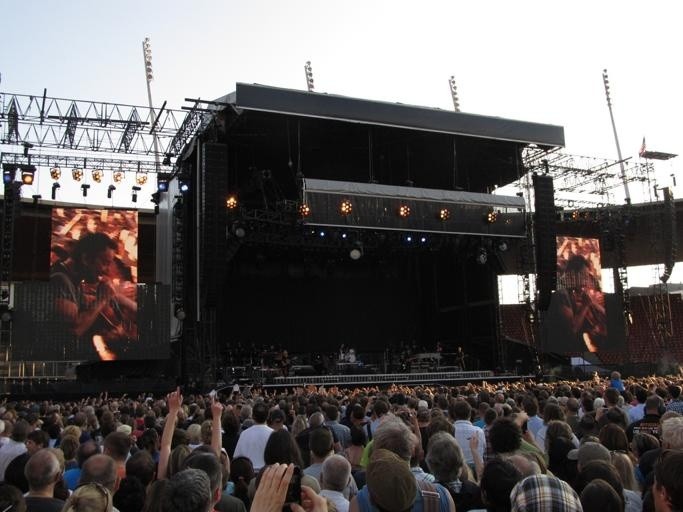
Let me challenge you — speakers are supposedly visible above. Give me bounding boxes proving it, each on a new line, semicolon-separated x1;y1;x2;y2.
533;176;556;311
200;143;228;307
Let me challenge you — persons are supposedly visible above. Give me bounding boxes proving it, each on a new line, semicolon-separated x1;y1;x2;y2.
338;343;356;364
0;371;682;512
456;347;465;371
436;342;443;353
49;230;137;360
281;350;292;377
542;254;608;368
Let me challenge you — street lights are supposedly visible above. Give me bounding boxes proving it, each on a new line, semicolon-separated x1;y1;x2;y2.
304;59;316;92
141;35;163;173
447;74;463;114
601;67;634;203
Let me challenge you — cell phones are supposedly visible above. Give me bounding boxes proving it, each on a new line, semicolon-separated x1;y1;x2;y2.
283;467;301;512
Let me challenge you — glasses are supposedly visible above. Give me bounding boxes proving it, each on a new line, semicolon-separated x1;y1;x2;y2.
75;480;110;512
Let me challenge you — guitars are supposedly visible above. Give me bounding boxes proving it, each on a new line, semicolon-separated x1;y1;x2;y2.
282;356;298;367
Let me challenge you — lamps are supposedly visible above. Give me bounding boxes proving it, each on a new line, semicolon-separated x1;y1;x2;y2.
350;243;362;260
1;163;189;193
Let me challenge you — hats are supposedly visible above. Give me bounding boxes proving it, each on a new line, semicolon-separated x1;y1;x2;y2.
366;449;416;512
567;442;611;462
510;474;583;511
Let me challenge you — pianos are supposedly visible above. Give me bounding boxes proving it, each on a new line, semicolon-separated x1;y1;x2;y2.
412;352;439;360
